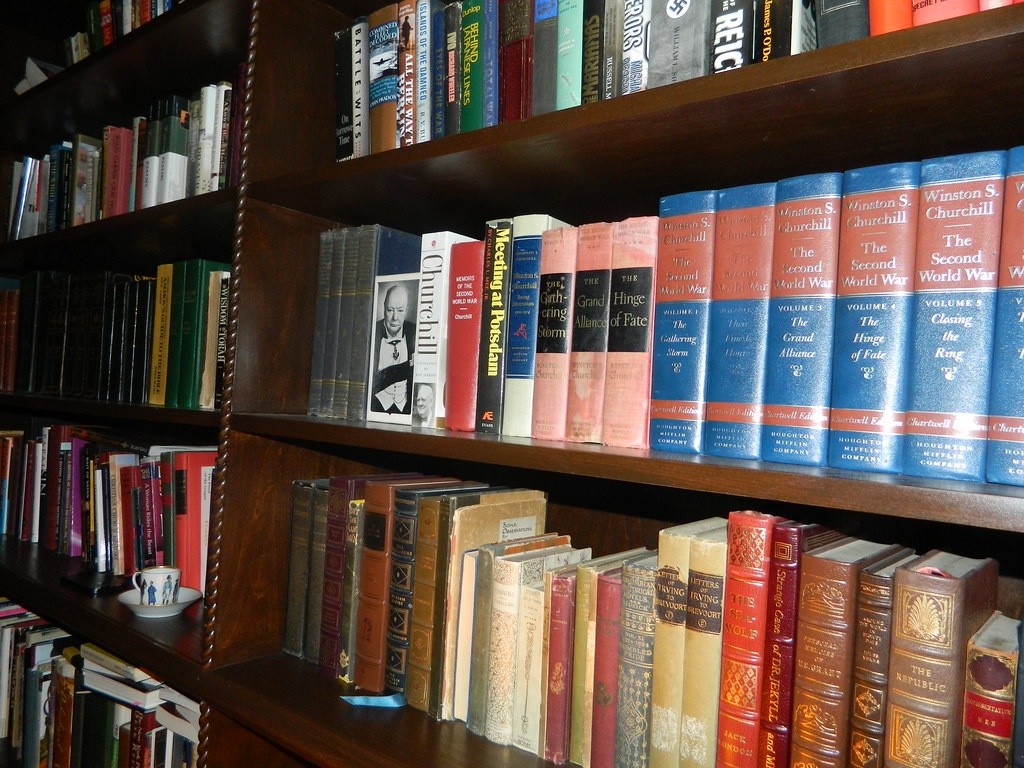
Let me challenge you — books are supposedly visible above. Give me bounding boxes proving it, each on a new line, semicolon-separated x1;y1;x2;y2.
0;0;1024;768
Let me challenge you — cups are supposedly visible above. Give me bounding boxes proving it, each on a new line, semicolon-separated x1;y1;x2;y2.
132;566;181;606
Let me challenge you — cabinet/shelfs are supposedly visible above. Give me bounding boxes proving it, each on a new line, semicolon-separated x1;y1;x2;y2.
0;0;1024;768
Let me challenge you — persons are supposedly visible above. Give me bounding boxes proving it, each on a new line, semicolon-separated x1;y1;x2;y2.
371;285;417;414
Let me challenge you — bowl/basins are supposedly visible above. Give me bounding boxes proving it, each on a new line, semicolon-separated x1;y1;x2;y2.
117;587;202;618
